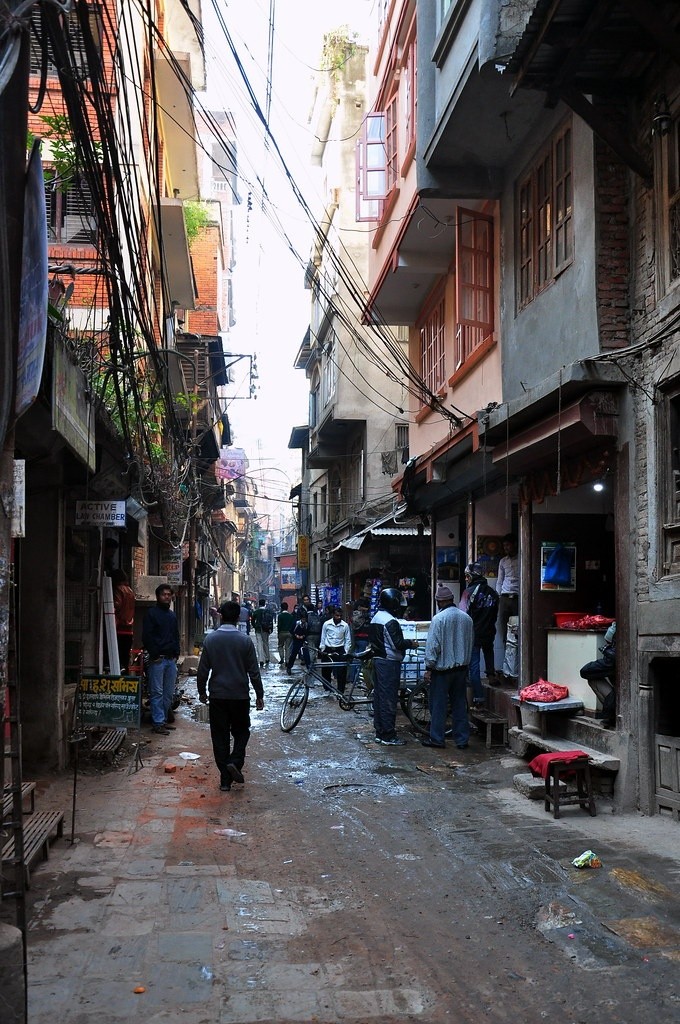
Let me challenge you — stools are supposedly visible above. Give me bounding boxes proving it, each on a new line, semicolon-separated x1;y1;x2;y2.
540;750;599;820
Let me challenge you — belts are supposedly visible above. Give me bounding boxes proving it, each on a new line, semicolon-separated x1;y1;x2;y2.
160;655;174;660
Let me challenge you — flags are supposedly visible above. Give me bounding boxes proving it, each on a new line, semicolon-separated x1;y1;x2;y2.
7;138;49;427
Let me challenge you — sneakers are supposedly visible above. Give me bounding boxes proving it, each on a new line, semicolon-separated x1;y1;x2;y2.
375;736;407;746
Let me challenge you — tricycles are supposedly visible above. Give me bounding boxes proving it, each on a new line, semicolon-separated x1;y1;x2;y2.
281;642;470;738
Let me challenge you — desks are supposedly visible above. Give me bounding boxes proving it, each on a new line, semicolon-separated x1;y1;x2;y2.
538;626;618;722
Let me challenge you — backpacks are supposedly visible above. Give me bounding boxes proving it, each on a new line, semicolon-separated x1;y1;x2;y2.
261;611;273;633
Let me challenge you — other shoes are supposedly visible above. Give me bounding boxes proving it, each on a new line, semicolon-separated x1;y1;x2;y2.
458;743;468;749
422;739;445;748
227;760;244;783
287;668;291;675
489;675;500;685
221;782;231;791
324;690;330;697
154;724;176;735
260;660;269;668
279;658;288;666
470;701;487;711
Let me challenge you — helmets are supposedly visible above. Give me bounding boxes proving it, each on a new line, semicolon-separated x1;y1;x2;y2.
380;588;407;610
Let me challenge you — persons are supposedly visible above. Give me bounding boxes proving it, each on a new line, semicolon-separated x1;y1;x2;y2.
141;584;181;735
350;604;371;683
368;588;420;746
579;621;618;727
110;566;135;675
319;607;351;700
352;593;371;611
197;601;265;791
421;587;474;748
456;562;501;712
496;534;519;674
235;595;332;676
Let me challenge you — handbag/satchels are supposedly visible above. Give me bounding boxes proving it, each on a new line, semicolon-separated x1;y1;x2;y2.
544;543;571;587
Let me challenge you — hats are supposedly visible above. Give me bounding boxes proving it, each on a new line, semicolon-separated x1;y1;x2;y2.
464;562;485;578
436;586;454;600
604;622;617;643
